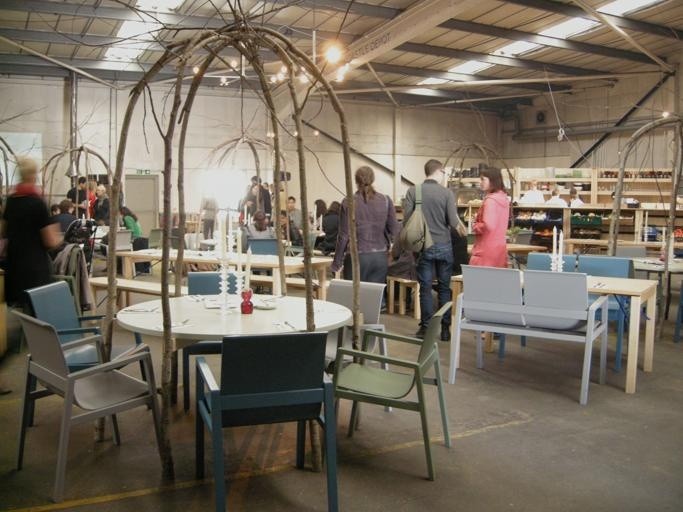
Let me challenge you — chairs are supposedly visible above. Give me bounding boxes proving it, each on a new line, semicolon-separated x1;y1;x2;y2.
11;224;454;511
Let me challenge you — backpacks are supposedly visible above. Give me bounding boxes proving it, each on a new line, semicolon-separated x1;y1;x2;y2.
399;207;434;254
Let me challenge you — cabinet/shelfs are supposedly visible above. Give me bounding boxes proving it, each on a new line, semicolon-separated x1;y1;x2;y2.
437;165;683;252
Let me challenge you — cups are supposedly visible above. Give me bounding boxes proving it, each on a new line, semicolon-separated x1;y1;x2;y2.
455;163;486;178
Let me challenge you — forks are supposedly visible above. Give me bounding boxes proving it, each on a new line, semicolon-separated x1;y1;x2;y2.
125;288;299;332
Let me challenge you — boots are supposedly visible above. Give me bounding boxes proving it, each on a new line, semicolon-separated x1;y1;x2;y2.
415;324;427;337
440;323;451;342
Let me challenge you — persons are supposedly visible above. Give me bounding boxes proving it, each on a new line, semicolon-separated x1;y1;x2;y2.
1;157;143;396
568;190;584;207
518;181;544;204
546;190;569;208
468;167;512;340
199;197;220;240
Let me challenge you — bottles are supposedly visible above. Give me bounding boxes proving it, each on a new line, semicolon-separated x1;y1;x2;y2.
540;182;590;191
603;170;672;178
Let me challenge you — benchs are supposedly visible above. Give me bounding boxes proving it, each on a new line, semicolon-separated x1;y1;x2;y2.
448;231;682;406
